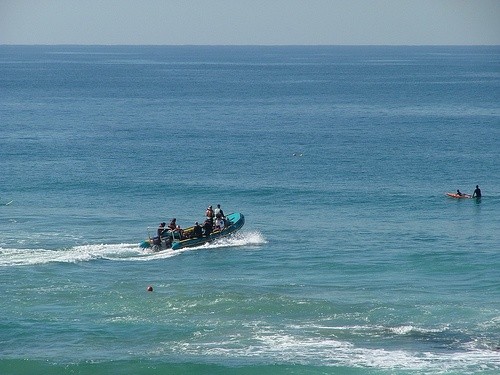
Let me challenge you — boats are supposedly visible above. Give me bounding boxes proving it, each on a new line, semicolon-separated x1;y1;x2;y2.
138;211;245;252
445;192;472;199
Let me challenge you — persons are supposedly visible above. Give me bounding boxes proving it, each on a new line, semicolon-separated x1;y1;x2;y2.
473;184;481;197
456;189;461;196
157;204;228;241
